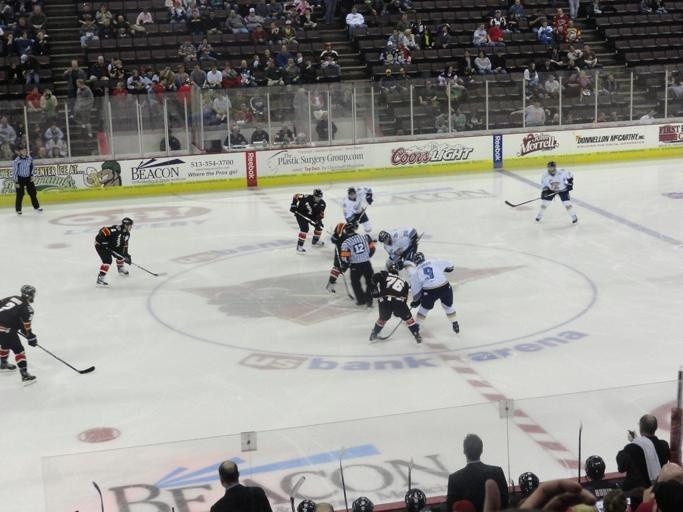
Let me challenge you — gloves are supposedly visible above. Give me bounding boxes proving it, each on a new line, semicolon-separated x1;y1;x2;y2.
566;185;572;190
371;289;379;298
15;182;20;188
26;334;36;346
366;193;373;205
290;204;297;212
541;191;548;199
124;254;131;265
351;218;358;229
30;176;35;182
410;301;420;308
105;247;111;252
315;221;323;230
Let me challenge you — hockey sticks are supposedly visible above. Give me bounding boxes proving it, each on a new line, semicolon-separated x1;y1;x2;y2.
378;305;412;344
358;202;370;224
505;184;568;208
17;329;96;376
109;247;166;280
297;211;333;238
339;271;353;302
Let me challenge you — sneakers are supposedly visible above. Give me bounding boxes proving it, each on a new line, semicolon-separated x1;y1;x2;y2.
97;278;108;285
37;208;42;211
297;246;305;252
372;238;377;242
572;215;577;222
370;331;377;340
366;300;372;307
329;283;335;292
312;240;324;245
118;267;129;274
410;325;418;336
22;374;36;380
535;215;543;220
356;302;366;305
18;211;22;214
0;362;16;369
453;322;459;333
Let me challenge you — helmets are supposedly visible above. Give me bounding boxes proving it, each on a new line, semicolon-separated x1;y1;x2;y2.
352;497;375;512
297;499;315;511
547;162;556;168
122;218;132;225
17;144;25;150
388;262;399;274
21;285;35;302
413;252;425;266
379;231;389;242
313;189;323;197
519;473;539;495
585;455;605;480
346;188;355;192
344;223;354;234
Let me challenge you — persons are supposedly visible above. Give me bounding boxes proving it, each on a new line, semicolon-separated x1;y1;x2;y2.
341;221;376;306
289;188;326;253
1;285;37;383
362;270;422;344
207;462;275;512
410;253;461;339
616;414;673;480
297;499;335;512
485;479;599;512
580;457;683;512
445;434;511;503
376;228;428;273
10;143;44;214
93;217;133;286
511;472;539;510
326;224;353;295
352;496;373;511
1;0;680;159
342;185;377;239
533;160;579;225
